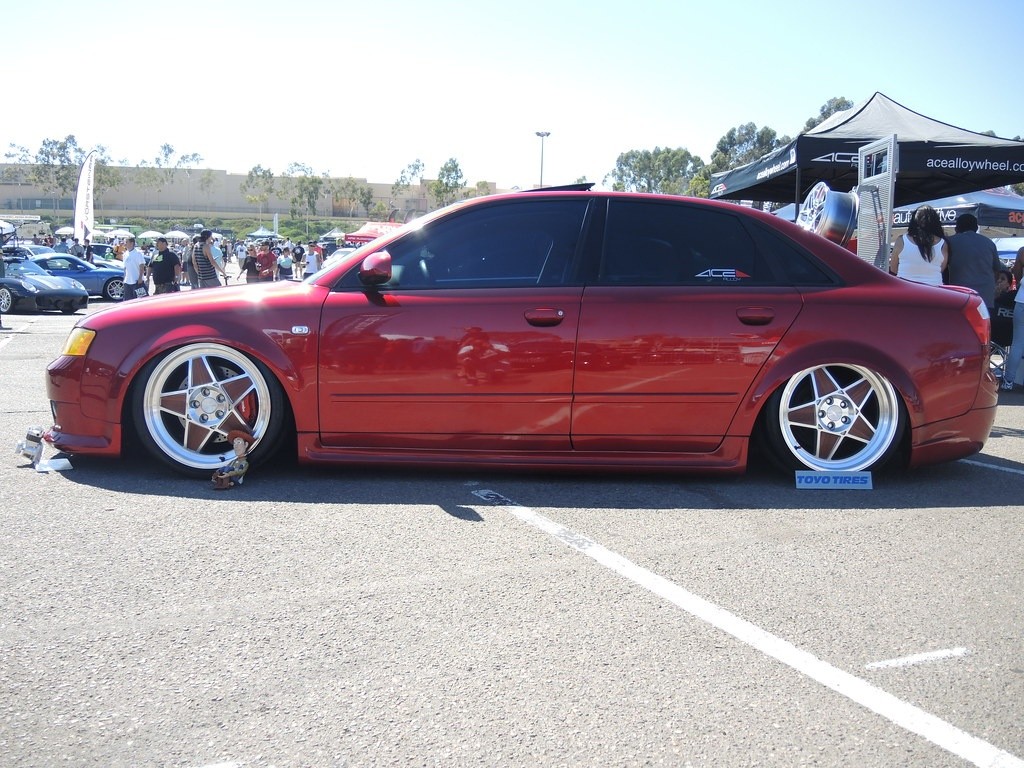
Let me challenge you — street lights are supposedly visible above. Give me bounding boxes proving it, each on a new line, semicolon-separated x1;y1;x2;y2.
534;131;551;188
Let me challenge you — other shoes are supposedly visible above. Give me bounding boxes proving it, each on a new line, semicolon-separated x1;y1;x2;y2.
1000;378;1014;392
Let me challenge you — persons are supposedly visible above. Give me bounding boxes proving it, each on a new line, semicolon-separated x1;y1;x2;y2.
890;206;1024;391
0;233;364;332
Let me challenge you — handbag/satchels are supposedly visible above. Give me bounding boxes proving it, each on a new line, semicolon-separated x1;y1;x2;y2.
135;281;149;298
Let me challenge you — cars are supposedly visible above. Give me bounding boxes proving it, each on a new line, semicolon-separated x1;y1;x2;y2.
0;225;145;316
44;182;998;483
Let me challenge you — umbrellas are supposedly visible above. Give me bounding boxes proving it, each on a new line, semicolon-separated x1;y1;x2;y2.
53;230;223;242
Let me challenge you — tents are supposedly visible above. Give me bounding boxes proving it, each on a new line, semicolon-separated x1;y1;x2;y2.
705;92;1024;229
247;222;408;243
